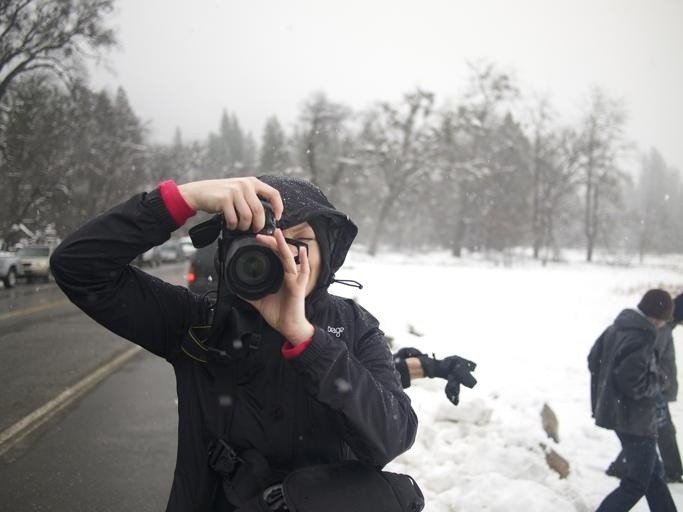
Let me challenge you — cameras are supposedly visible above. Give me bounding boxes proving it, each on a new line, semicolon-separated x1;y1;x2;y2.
217;208;284;301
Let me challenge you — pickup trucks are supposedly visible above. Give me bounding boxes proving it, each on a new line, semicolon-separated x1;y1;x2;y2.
0;250;18;288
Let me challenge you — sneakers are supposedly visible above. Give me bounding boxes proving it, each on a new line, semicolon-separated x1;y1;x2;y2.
605;462;622;479
661;471;683;484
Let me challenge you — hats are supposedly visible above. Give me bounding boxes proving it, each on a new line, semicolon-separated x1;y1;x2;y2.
638;289;673;321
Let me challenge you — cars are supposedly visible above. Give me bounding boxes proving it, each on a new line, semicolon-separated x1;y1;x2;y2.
131;237;198;268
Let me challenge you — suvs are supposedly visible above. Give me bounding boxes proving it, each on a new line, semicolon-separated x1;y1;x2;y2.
185;236;222;300
15;245;56;282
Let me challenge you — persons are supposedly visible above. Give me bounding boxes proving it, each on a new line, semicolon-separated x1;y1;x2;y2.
576;288;680;511
599;292;682;485
48;171;424;511
